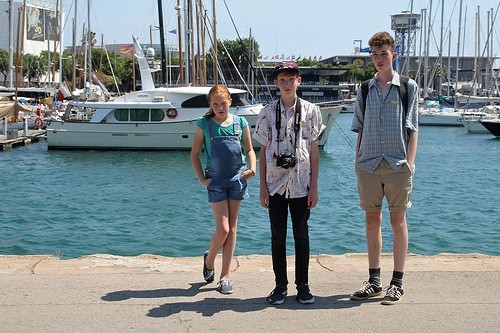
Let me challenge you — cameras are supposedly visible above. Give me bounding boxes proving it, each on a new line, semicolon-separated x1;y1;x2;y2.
276;150;297;169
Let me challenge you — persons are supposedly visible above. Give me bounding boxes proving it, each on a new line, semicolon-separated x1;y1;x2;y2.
190;85;256;294
252;63;327;305
351;32;420;306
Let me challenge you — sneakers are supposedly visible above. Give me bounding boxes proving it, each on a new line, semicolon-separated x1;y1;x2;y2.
296;284;314;304
351;280;383;300
268;285;287;304
203;251;215;283
217;279;234;294
382;285;405;304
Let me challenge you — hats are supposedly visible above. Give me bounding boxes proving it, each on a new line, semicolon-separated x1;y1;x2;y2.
273;62;299;75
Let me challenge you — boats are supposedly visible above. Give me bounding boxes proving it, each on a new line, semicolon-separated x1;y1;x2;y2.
478;119;500;137
417;107;476;124
0;102;81;131
46;35;344;150
458;112;499;133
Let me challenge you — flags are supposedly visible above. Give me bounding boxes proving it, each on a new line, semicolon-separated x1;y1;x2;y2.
119;45;134;55
257;54;322;62
